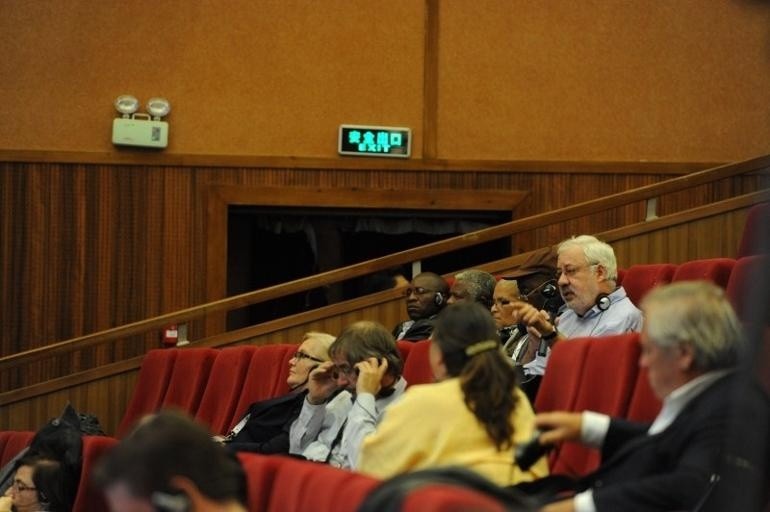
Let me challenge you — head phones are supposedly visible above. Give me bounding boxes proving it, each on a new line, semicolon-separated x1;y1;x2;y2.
332;348;396;378
434;290;447;307
542;281;610;311
149;413;191;512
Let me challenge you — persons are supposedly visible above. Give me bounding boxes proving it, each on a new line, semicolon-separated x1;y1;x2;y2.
534;280;770;512
513;233;647;378
499;246;561;383
286;320;410;471
448;269;494;311
0;451;72;512
390;272;449;344
488;278;524;326
90;409;253;512
358;304;549;490
211;328;338;454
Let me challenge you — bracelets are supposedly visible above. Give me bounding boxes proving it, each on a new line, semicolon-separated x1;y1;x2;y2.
538;327;558;341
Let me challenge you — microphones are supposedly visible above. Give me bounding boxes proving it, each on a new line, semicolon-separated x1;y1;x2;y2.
288;365;318;394
538;294;560;356
12;490;49;510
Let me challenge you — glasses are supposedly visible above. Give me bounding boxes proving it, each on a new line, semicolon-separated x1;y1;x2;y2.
331;368;355;378
404;289;432;296
293;350;325;365
9;482;36;493
555;261;598;277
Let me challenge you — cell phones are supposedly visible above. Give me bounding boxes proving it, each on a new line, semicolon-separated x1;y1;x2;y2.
514;427;553;471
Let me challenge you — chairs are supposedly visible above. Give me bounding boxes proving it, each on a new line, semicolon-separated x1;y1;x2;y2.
112;324;763;495
738;205;769;257
433;252;769;337
0;429;506;511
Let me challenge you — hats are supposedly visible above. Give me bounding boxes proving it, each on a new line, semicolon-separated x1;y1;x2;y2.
500;245;558;280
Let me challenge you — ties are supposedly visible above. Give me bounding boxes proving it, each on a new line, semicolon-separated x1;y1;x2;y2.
322;418;348;463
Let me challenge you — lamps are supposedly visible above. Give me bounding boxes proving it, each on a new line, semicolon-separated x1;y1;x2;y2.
105;89;173;149
